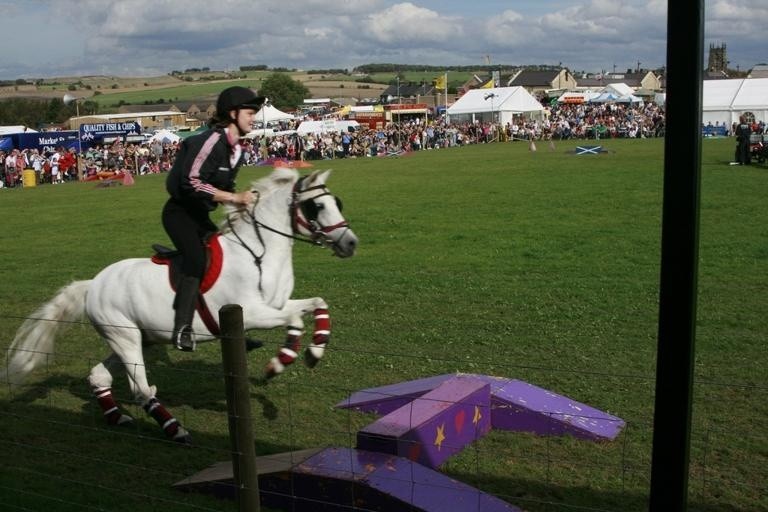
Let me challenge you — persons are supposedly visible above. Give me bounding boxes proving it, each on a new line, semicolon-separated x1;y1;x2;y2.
735;116;754;165
244;121;502;165
1;131;184;187
162;85;273;351
702;119;728;129
506;100;667;140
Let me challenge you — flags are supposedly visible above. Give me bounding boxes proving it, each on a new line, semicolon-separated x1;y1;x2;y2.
433;72;448;91
480;77;495;89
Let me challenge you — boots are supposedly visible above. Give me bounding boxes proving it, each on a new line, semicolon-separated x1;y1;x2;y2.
172;277;202;351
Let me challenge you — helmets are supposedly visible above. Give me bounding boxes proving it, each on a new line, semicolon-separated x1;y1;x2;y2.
217;86;266;119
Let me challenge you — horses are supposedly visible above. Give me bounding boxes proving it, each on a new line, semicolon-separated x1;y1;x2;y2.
0;167;360;449
